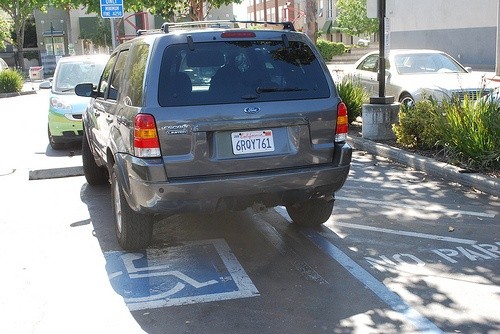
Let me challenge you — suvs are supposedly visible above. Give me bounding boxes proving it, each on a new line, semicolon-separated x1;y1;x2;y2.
73;18;354;252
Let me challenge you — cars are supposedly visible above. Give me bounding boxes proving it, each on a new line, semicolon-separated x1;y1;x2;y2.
336;48;499;114
38;53;112;150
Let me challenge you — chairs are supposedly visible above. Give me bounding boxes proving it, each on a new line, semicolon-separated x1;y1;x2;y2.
209;52;255;94
158;54;192;94
